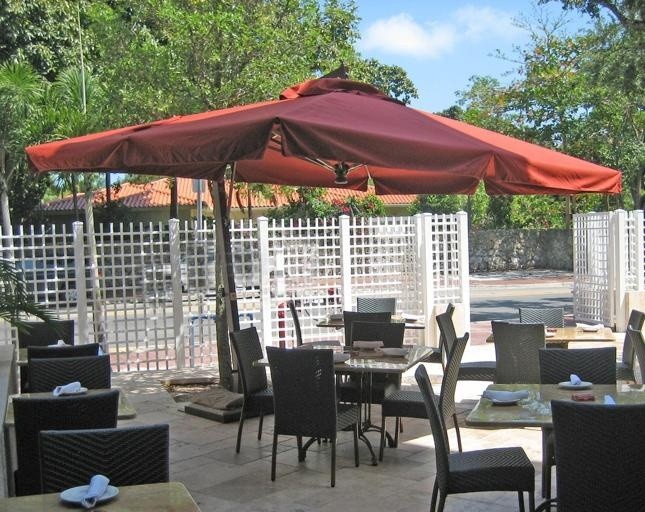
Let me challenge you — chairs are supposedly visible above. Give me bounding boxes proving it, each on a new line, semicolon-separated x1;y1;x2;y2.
378;332;471;465
333;321;412;451
488;317;547;383
437;312;497;382
341;309;391;347
518;305;566;328
537;346;617;512
228;324;326;458
427;301;457;372
357;296;396;314
615;308;643;379
545;397;645;511
0;312;202;512
627;322;644;375
412;362;540;512
262;346;363;489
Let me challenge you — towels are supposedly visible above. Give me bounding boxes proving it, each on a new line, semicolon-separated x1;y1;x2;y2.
604;394;615;406
481;389;528;401
570;374;582;385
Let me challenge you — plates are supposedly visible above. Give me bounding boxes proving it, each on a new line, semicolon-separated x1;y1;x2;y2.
491;398;519;405
558;381;594;388
404;318;417;322
581;326;600;331
65;386;88;395
58;485;119;502
329;314;344;320
333;353;350;363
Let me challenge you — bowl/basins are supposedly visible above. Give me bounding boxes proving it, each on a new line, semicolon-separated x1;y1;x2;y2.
380;348;410;355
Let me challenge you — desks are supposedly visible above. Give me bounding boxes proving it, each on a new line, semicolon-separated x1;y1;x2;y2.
463;380;645;511
318;312;426;335
485;321;615;353
252;341;437;468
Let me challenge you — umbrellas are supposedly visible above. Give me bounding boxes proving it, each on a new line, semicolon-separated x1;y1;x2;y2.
24;62;623;323
